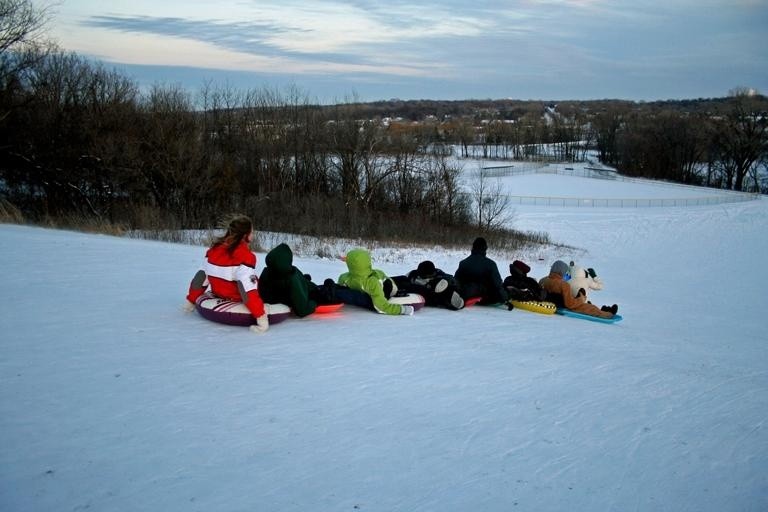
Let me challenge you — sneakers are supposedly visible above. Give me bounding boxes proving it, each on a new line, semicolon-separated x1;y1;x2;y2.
601;304;618;315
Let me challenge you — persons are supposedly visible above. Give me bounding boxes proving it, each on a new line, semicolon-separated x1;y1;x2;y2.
404;260;472;311
179;215;336;332
565;259;603;304
537;259;618;319
337;248;446;318
504;260;563;311
455;237;535;311
258;243;393;317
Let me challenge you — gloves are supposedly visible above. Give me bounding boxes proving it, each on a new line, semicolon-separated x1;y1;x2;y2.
504;301;514;311
249;313;270;333
179;299;195;312
588;268;597;278
400;306;414;315
